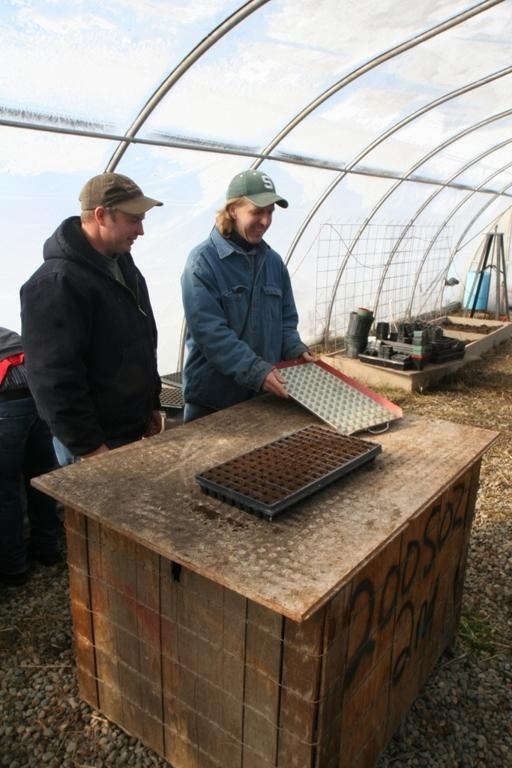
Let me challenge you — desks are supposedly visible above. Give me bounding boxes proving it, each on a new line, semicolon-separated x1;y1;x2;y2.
30;391;500;768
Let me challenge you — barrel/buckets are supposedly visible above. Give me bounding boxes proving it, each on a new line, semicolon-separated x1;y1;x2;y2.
346;312;375;359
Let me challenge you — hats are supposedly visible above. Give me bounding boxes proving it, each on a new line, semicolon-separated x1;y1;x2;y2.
226;168;291;209
78;172;166;217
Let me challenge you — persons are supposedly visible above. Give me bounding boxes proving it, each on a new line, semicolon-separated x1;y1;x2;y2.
179;169;319;423
1;328;64;585
19;172;164;465
